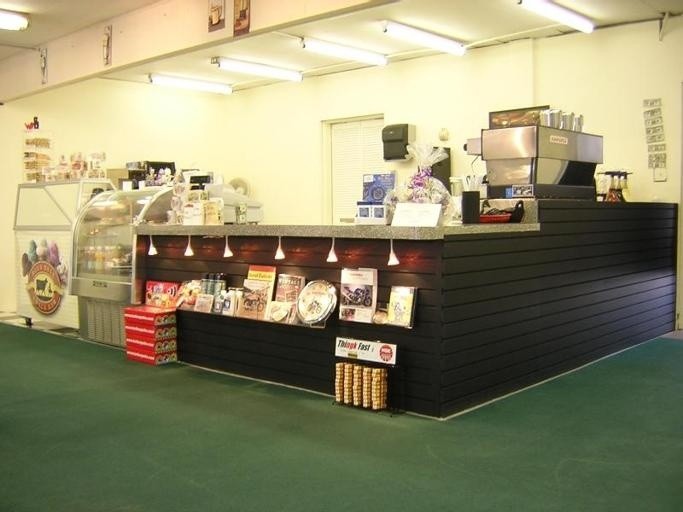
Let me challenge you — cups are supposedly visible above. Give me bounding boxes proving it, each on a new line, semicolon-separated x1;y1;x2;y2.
234;202;248;225
461;191;480;224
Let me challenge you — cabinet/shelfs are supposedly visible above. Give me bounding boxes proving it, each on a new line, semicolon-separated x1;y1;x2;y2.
69;186;172;303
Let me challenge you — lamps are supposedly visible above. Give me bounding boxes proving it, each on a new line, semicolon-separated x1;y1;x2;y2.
150;71;234;94
0;9;32;33
514;0;594;36
147;236;401;266
206;58;305;86
298;35;390;69
377;19;469;59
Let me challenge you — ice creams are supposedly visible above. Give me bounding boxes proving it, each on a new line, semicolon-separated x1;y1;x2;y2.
56;261;68;288
20;253;32;283
37;239;49;262
28;240;36;264
48;240;61;269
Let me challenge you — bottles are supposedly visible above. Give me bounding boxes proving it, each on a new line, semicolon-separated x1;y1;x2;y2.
79;243;129;275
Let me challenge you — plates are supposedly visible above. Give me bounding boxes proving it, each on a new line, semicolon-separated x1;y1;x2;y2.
480;212;512;223
